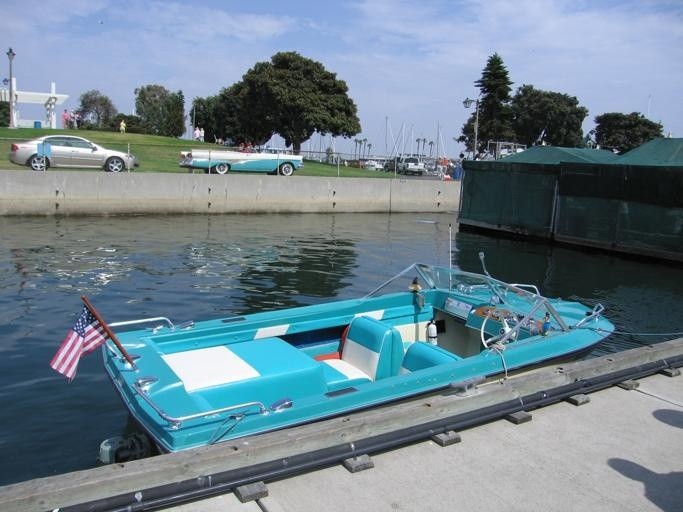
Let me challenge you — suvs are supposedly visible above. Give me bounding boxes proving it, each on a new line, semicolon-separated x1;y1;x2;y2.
384;157;404;174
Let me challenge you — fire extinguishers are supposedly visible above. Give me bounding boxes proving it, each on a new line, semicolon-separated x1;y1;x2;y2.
427;317;438;346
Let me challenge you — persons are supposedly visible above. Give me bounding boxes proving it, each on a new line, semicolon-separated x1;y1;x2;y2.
437;155;442;175
75;111;81;130
474;153;480;160
119;119;128;135
443;157;448;174
458;154;467;179
69;110;74;129
63;108;70;128
194;126;200;140
200;127;206;144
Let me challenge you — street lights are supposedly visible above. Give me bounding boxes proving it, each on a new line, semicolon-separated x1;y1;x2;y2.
462;97;479;160
7;46;15;127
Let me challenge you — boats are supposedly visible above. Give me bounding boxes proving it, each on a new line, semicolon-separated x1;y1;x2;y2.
97;262;616;464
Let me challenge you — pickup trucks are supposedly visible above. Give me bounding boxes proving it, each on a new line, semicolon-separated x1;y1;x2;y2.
396;157;425;176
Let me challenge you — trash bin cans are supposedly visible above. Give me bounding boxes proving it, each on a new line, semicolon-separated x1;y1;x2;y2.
34;121;42;128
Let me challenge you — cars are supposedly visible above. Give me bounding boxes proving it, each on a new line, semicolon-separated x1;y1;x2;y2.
178;147;303;177
350;160;384;171
10;135;135;172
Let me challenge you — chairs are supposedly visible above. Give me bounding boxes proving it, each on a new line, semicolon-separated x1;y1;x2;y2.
317;316;461;383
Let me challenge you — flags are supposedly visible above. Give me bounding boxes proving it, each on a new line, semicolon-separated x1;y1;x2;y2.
48;305;110;382
